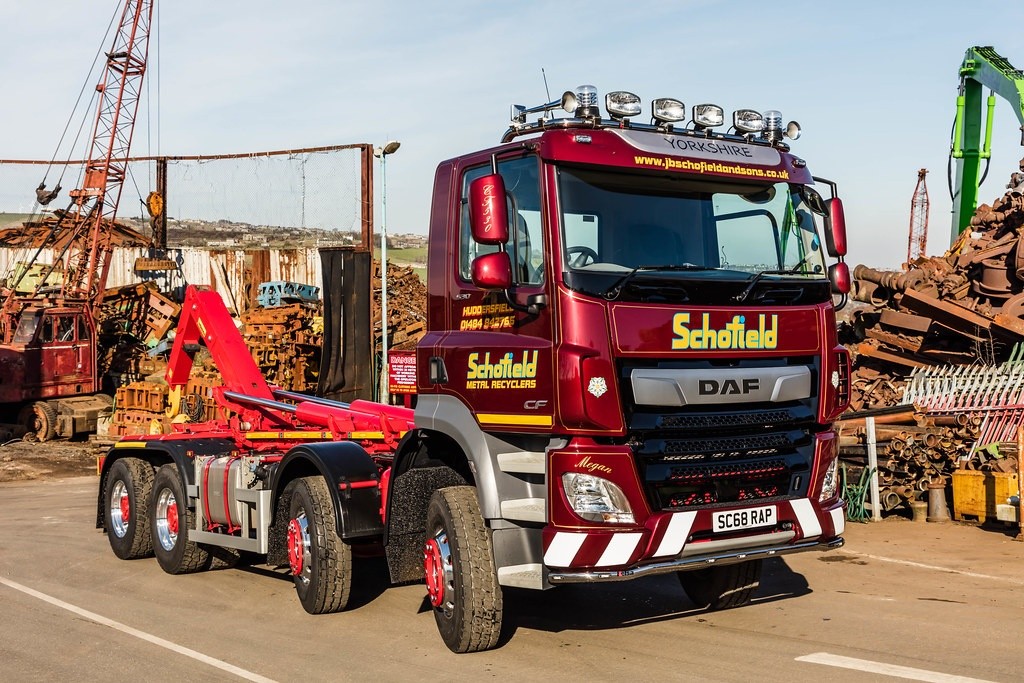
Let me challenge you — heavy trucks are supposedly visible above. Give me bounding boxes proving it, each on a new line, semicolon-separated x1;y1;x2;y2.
94;84;852;655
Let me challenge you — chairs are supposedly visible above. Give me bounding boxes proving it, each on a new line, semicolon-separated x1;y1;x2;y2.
617;224;684;270
480;210;538;285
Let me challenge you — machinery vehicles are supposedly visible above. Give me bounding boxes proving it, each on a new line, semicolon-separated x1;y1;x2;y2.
0;0;165;443
